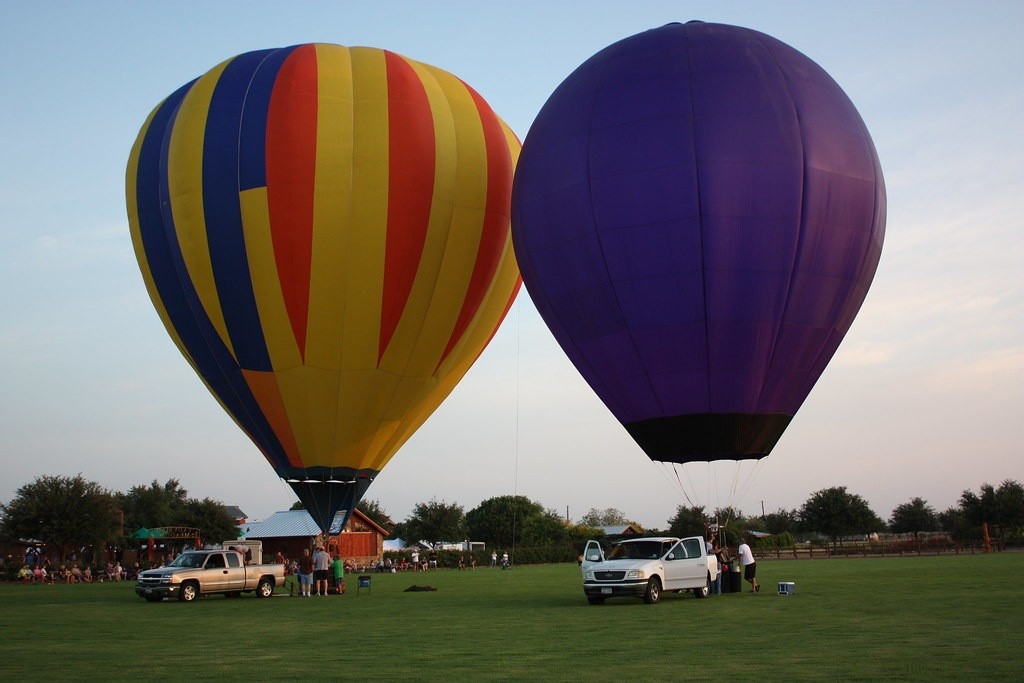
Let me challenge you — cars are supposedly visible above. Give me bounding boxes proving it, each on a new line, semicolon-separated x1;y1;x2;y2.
581;534;722;605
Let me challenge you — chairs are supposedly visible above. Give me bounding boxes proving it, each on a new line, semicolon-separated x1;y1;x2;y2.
357;576;371;595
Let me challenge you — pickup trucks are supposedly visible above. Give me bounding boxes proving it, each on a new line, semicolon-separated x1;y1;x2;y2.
135;549;288;603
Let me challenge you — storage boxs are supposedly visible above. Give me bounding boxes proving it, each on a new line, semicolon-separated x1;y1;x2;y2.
778;581;794;595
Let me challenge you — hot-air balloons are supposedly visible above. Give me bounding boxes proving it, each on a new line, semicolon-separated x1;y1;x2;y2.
118;42;524;598
509;17;890;594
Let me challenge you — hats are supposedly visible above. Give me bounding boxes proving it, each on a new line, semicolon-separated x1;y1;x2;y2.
319;546;325;550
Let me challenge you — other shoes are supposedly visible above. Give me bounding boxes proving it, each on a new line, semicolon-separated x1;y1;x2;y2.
755;582;760;591
290;590;343;597
750;590;754;593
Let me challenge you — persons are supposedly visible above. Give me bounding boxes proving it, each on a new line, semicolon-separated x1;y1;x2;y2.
706;533;729;595
458;555;476;570
229;543;252;566
578;554;583;566
343;549;438;574
491;550;497;567
296;548;344;597
730;537;760;593
133;554;174;580
277;552;284;564
104;562;127;582
502;551;508;564
18;564;56;585
60;564;93;584
